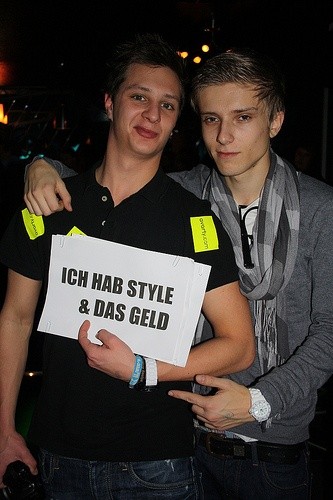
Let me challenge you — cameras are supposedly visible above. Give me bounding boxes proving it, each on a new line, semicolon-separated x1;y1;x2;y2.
4;461;50;500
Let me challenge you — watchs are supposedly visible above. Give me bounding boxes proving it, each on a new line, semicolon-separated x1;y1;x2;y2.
247;387;271;422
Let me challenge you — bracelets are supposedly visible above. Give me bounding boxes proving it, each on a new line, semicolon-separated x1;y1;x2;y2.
144;357;159;392
129;355;144;389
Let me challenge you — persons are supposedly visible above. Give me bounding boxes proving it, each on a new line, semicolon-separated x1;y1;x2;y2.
166;46;333;500
0;45;256;489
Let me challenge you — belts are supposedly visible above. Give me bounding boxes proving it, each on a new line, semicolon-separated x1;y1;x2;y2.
194;426;308;465
